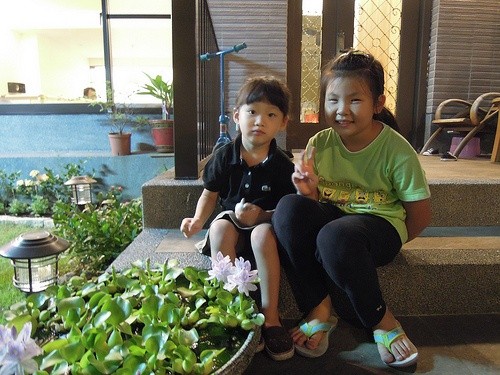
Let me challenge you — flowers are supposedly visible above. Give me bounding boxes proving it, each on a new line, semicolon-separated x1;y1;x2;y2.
0;251;265;375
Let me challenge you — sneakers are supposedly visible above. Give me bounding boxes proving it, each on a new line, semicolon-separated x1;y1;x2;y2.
260;317;294;360
422;147;439;156
441;152;457;160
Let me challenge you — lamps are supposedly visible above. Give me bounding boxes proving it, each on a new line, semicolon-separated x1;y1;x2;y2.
0;230;70;297
62;175;97;212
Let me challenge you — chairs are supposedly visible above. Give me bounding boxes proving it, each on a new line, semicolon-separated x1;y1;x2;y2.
419;93;500;161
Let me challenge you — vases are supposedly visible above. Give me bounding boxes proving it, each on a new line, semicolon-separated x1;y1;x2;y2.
211;296;262;375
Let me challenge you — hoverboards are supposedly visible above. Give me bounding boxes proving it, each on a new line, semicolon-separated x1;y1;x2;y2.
200;42;248;156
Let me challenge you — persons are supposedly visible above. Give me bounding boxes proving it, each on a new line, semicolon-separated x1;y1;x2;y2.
179;77;298;360
271;50;431;367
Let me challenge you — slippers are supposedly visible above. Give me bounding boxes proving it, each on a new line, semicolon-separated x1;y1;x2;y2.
373;320;418;367
293;315;338;358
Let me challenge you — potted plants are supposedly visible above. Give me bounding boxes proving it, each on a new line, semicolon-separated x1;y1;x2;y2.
87;70;143;156
137;72;174;153
